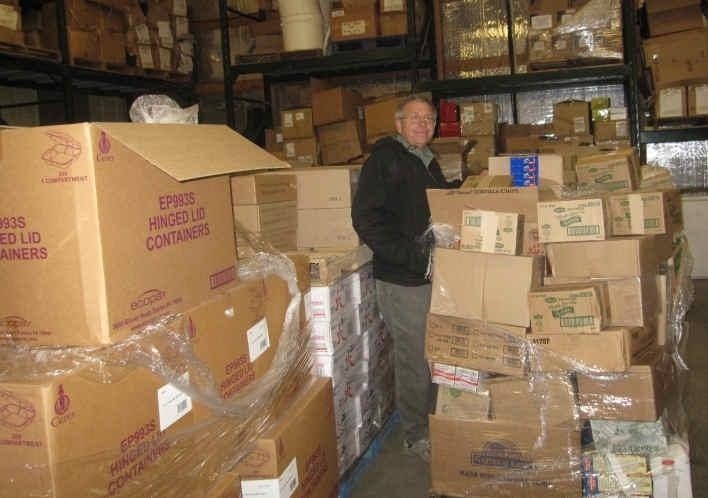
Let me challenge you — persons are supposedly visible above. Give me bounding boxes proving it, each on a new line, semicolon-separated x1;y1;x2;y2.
350;96;488;464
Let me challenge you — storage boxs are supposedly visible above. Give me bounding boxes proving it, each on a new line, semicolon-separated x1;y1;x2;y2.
1;123;397;498
425;124;695;498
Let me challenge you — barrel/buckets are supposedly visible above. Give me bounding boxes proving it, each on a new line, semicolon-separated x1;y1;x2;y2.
278;0;322;52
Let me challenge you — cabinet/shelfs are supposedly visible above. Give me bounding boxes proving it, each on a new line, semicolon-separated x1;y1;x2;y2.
438;0;708;194
5;1;437;153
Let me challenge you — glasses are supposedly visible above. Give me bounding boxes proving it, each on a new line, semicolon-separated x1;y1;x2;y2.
400;113;436;122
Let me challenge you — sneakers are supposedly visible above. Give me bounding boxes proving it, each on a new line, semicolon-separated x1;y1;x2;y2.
401;438;434;463
